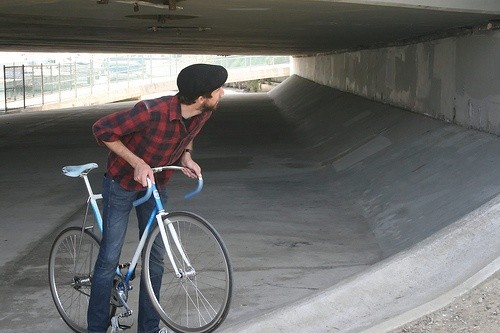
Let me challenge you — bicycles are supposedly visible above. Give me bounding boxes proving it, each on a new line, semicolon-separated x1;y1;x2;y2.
47;159;233;333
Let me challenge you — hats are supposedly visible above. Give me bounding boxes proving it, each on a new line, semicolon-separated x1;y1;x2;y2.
177;64;228;97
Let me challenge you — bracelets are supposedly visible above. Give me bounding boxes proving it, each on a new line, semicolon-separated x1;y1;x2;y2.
183;149;192;153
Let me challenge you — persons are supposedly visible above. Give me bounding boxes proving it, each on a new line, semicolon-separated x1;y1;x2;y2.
85;64;228;333
